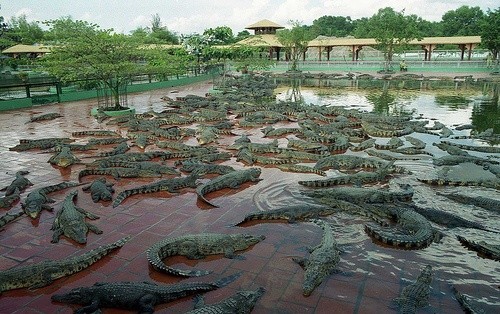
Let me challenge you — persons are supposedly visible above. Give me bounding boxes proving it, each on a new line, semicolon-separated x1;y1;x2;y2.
486;52;493;68
400;59;407;71
